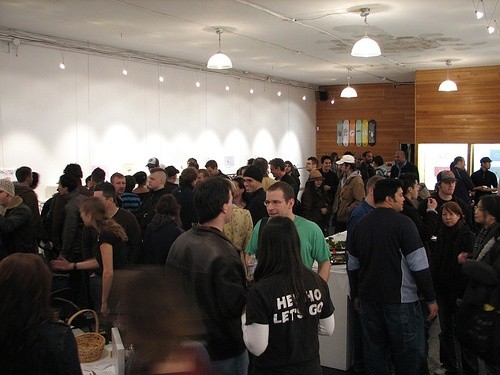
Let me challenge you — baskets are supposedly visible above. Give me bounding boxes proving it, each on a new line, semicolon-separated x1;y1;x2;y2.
66;310;105;363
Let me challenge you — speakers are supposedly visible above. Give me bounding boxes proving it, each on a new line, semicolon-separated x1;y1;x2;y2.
319;91;327;101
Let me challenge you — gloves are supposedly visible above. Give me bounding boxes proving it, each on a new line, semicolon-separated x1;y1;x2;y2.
187;157;197;164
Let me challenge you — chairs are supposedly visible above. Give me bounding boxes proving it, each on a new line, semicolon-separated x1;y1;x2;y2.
53;298;96;332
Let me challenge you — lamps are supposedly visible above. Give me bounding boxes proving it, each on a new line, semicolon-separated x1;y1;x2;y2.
207;32;231;69
439;63;457;91
351;9;381;57
340;69;358;98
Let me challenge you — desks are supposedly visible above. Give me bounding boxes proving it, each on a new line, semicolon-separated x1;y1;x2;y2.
311;230;359;370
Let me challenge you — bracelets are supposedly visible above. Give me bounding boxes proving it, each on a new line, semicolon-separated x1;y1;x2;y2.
73;263;77;269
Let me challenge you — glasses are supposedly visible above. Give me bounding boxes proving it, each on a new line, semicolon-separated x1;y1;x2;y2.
263;198;286;207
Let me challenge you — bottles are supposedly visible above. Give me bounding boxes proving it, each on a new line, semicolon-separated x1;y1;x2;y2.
247;254;258;276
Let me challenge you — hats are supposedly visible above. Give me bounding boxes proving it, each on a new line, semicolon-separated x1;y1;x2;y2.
145;157;159;167
0;179;15;197
435;170;458;184
308;170;325;182
336;154;357;165
242;164;263;182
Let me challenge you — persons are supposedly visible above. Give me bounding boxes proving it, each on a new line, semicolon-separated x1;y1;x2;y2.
165;177;248;375
0;252;82;375
343;156;500;375
245;182;332;282
241;216;336;375
118;266;210;375
0;151;418;341
